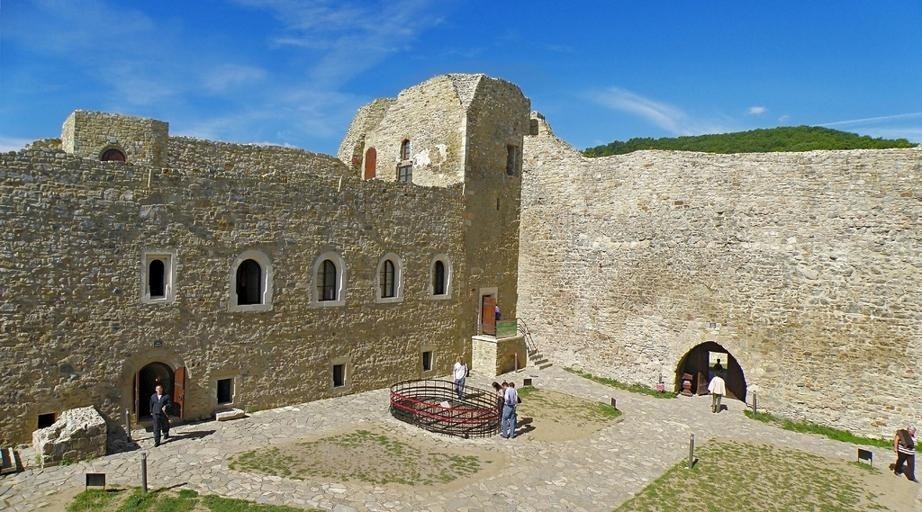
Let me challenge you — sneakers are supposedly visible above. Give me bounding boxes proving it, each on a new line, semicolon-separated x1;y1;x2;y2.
500;433;514;439
154;431;168;448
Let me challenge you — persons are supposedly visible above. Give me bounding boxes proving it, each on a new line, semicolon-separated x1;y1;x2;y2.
492;382;503;405
149;385;173;447
501;382;518;439
453;355;470;402
893;423;920;484
707;369;726;414
713;359;723;370
497;382;511;430
